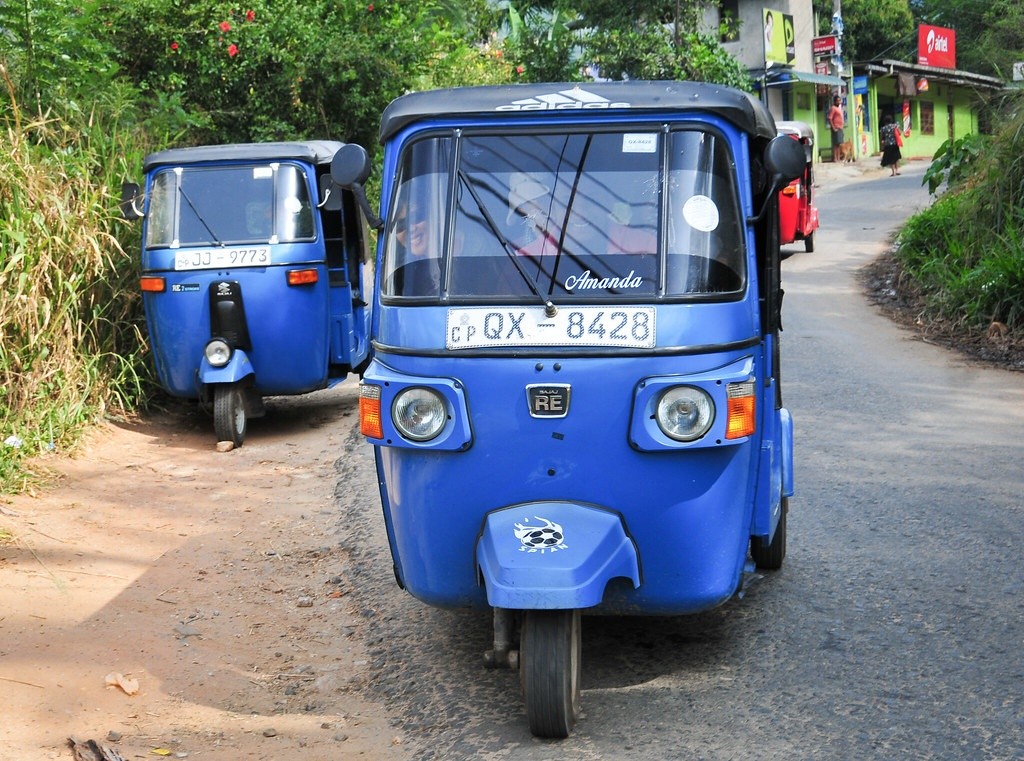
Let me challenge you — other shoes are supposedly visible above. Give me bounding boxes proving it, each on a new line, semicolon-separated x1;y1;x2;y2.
896;172;901;175
891;174;894;176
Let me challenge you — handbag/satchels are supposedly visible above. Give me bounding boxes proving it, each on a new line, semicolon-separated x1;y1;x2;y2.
894;128;903;147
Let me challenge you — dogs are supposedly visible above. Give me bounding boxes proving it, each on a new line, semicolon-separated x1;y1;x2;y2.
834;137;853;163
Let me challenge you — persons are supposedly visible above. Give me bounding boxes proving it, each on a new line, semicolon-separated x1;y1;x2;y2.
763;11;773;52
880;115;901;176
515;137;658;256
397;198;485;264
827;96;845;162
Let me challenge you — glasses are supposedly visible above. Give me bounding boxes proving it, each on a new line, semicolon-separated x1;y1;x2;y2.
389;207;428;235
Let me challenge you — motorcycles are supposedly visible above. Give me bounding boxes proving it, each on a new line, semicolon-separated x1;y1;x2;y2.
119;138;377;447
768;119;820;253
330;80;795;740
